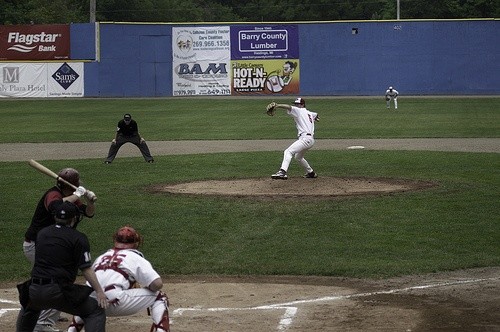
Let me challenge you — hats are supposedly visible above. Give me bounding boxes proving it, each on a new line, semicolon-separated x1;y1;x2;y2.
56;203;77;219
389;87;393;90
292;98;305;106
124;114;131;120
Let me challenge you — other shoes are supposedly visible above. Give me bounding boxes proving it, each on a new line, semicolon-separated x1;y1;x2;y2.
105;161;110;165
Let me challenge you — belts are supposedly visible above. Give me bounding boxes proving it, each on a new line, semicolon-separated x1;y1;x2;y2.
25;239;31;243
31;276;60;285
105;286;115;292
300;133;312;136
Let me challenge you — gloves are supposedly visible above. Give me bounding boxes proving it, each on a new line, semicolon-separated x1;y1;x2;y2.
72;186;86;197
84;190;96;203
112;298;120;307
140;137;145;144
112;139;116;144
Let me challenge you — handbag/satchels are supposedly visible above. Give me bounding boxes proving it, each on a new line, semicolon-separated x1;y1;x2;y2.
16;279;31;309
61;282;94;305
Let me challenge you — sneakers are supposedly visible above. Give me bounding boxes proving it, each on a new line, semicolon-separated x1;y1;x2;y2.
271;171;288;179
34;324;63;332
301;172;318;178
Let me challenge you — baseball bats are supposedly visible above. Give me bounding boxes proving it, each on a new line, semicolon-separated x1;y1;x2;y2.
28;158;98;202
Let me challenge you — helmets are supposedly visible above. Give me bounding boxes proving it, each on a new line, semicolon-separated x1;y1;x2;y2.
114;226;137;248
58;167;80;192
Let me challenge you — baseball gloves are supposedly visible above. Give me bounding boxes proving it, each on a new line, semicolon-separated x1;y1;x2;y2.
266;101;277;116
386;98;390;101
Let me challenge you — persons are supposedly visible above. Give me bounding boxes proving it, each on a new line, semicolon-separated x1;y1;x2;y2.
266;98;320;179
386;86;399;110
104;114;154;165
23;168;97;332
17;200;106;332
66;227;170;332
